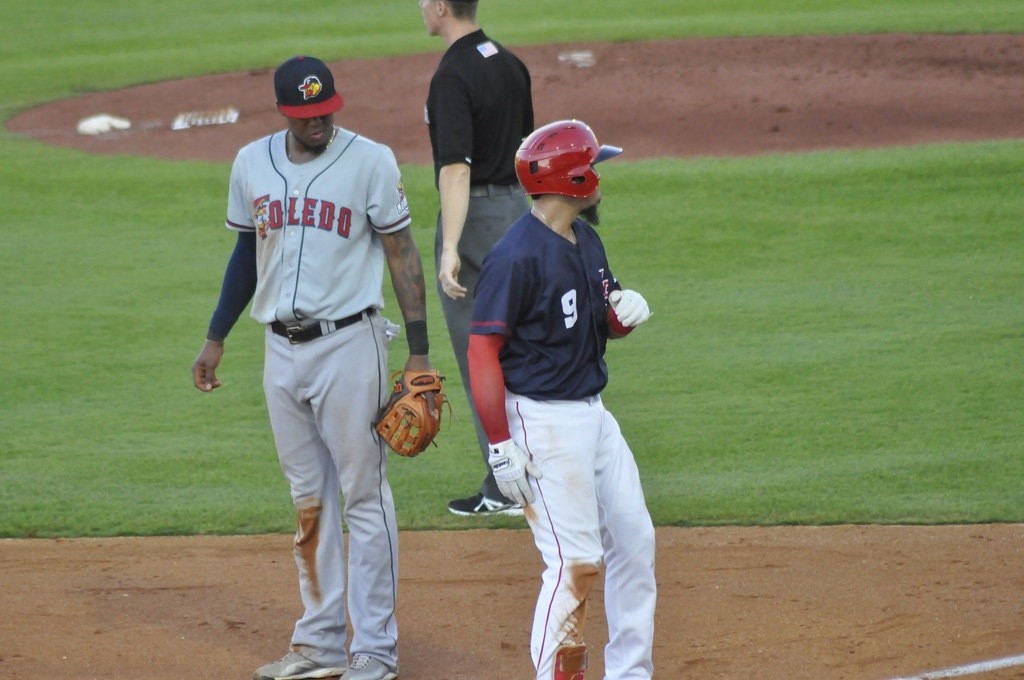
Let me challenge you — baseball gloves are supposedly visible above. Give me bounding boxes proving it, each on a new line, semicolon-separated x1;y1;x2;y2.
373;369;445;459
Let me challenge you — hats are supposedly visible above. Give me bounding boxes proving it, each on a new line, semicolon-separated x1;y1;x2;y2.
273;55;343;118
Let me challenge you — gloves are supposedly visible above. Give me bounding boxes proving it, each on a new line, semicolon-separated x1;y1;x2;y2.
610;289;654;329
488;439;541;506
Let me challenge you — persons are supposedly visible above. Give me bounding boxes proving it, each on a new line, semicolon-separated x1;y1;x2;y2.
420;0;534;517
190;53;443;680
467;119;656;680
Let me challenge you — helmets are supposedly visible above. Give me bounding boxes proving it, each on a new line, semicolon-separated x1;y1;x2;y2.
515;119;623;199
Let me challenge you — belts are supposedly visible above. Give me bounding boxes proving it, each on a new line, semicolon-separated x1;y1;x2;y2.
271;308;376;344
467;184;522;197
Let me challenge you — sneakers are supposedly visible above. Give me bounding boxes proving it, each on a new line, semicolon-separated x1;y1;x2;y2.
448;488;525;517
255;644;346;680
340;653;396;680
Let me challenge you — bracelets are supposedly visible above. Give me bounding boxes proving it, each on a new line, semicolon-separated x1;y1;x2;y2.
404;319;430;356
